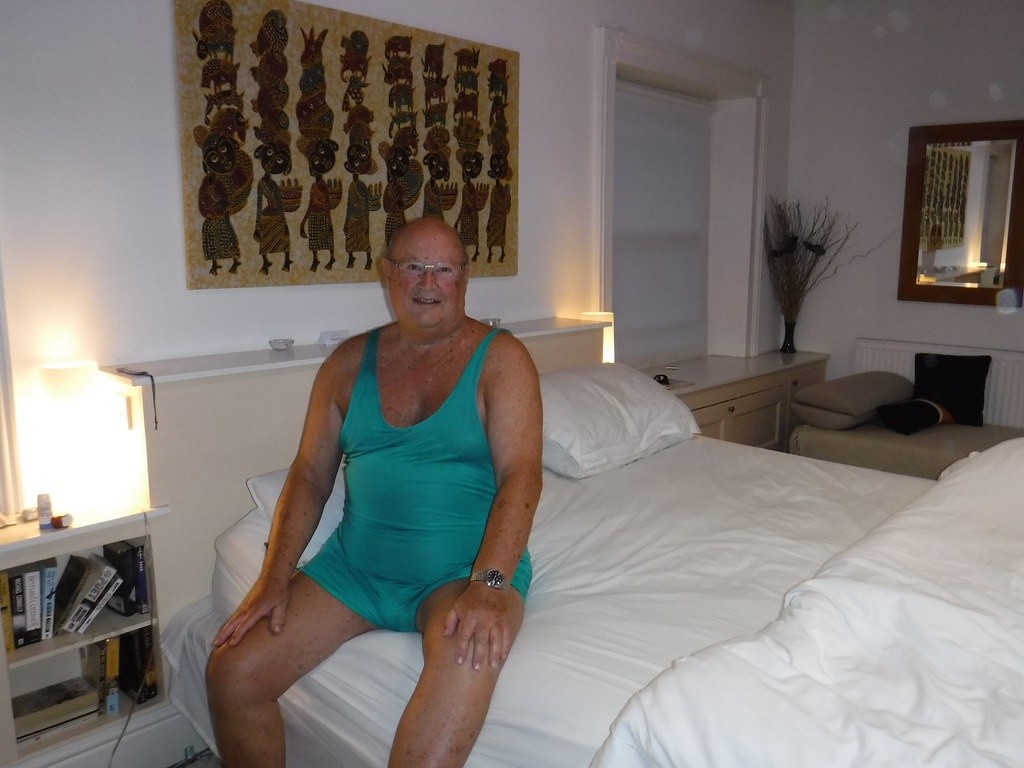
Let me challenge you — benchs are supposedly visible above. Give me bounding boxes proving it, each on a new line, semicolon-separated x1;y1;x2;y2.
788;425;1022;479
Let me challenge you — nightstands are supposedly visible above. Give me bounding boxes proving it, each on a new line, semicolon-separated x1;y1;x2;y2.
629;353;836;452
0;507;170;768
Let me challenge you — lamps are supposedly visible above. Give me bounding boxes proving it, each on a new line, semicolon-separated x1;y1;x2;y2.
583;312;615;365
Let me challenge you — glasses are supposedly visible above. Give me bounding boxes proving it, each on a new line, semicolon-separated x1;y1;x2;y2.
388;257;466;280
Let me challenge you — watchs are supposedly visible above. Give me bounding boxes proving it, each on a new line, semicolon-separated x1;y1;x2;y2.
468;567;508;590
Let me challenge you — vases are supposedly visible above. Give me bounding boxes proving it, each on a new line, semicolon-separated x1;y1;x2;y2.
779;318;795;354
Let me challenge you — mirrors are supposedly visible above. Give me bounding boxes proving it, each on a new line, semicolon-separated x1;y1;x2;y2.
897;121;1023;307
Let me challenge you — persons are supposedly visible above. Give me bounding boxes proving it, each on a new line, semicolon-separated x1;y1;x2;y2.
205;219;544;768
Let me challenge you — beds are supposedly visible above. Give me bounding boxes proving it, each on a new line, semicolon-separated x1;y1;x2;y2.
100;314;1022;766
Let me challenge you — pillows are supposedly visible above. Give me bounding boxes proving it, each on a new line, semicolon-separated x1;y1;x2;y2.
245;462;346;564
790;401;877;426
543;360;702;482
914;349;993;426
795;370;912;416
874;399;954;434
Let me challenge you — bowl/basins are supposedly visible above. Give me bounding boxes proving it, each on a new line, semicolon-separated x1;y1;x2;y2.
269;338;295;350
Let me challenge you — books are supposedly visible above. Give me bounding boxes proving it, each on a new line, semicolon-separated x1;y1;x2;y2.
12;675;101;746
0;539;151;664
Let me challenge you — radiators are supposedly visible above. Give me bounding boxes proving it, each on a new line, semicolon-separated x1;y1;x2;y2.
849;339;1024;427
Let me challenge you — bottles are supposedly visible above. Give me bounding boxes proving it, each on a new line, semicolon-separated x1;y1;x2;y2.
38;493;52;531
